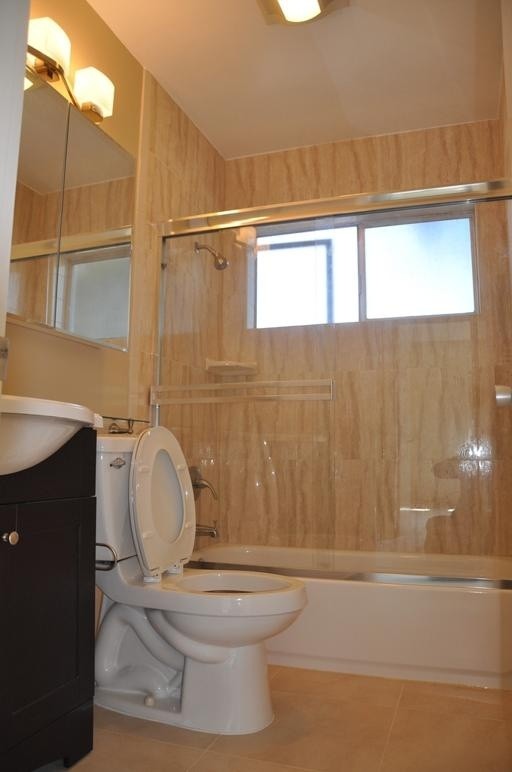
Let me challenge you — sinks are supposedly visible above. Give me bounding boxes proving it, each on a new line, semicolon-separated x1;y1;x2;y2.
0;393;104;475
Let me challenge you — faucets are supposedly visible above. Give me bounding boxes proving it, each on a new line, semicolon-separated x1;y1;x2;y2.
197;527;217;538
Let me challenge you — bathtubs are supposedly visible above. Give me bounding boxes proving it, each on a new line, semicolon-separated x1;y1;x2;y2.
181;542;511;693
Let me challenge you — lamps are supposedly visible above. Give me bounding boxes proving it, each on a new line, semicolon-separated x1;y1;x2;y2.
25;15;116;127
231;228;261;248
255;1;349;29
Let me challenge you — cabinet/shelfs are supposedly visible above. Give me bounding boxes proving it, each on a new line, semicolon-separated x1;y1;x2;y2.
0;495;99;771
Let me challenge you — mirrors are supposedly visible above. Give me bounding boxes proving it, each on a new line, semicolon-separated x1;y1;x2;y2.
7;72;140;352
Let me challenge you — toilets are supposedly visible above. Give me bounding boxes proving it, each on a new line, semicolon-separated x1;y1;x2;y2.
97;420;307;736
